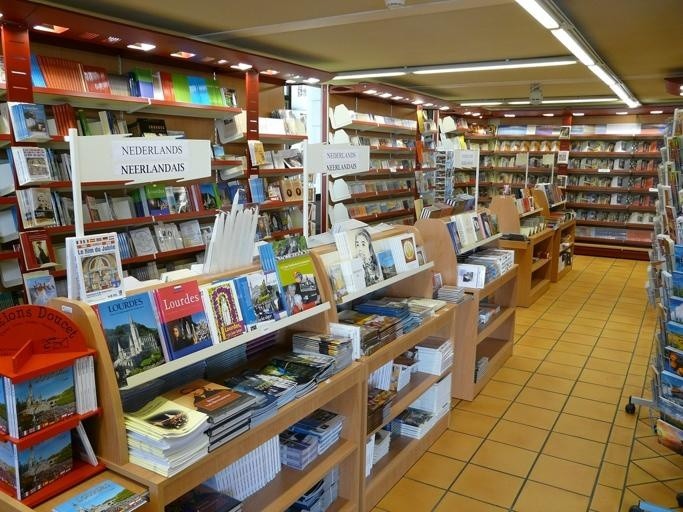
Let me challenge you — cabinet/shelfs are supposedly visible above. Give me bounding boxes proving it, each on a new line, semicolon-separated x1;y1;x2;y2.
0;1;683;511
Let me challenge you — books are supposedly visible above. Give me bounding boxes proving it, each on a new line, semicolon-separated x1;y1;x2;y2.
417;108;649;242
384;232;465;439
648;109;681;450
3;58;417;508
479;291;503;324
457;249;515;288
474;356;491;382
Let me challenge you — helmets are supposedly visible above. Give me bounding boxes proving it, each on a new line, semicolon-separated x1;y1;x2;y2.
291;270;301;278
